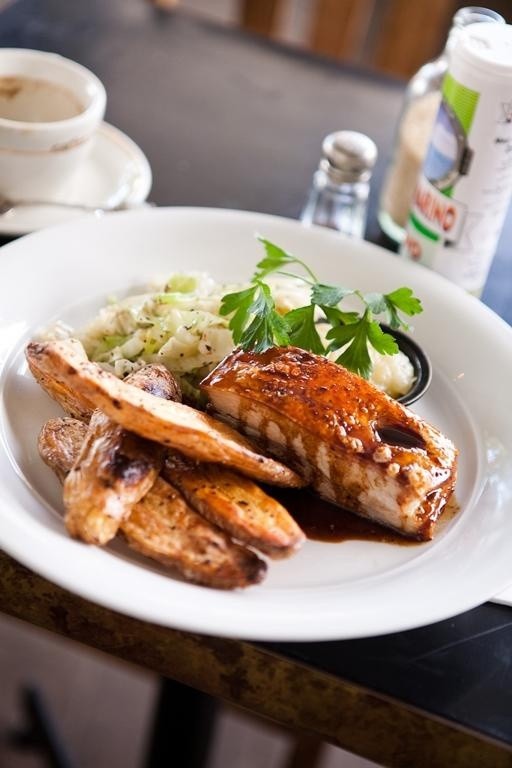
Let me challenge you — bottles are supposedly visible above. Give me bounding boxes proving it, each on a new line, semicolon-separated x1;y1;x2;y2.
376;6;512;241
298;130;378;240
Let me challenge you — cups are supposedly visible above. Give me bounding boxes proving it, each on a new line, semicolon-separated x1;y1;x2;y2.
0;47;108;213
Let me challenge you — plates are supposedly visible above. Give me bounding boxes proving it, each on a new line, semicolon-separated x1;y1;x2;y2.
0;120;154;237
0;206;512;643
